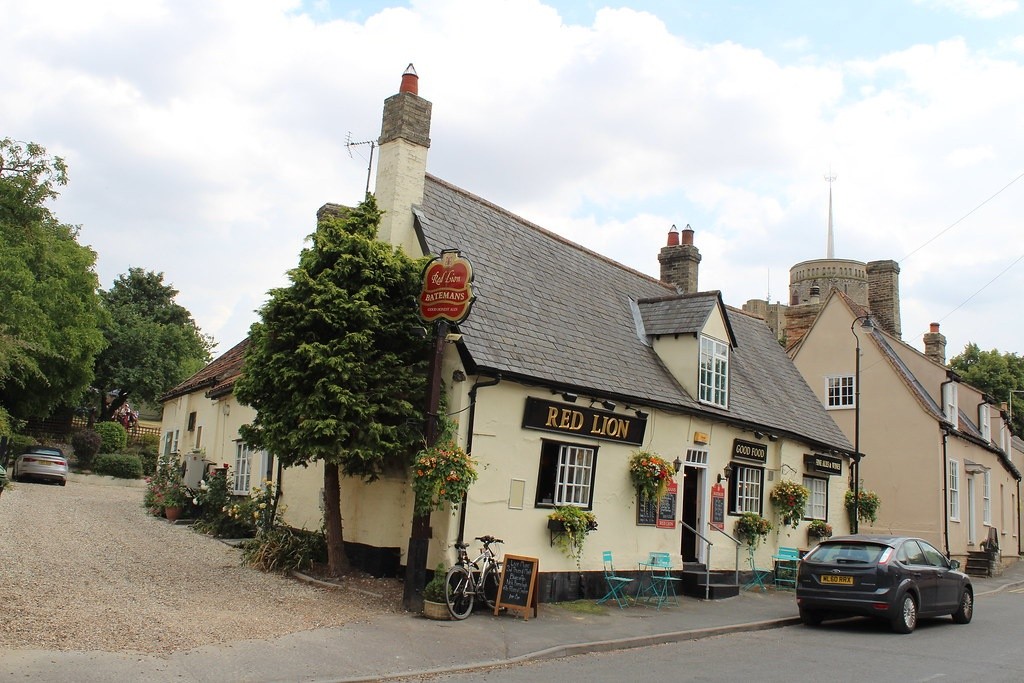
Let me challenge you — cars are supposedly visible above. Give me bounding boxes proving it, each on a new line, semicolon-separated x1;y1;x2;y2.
12;445;68;486
794;534;974;634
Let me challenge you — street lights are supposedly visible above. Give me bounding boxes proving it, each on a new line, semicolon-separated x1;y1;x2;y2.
851;312;875;534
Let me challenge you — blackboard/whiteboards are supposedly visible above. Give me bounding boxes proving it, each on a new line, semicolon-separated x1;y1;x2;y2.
496;554;539;611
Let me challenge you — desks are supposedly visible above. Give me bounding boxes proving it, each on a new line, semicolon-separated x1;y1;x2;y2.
771;555;801;589
638;560;673;611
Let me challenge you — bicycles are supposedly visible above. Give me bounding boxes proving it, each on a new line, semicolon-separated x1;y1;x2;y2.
444;535;505;621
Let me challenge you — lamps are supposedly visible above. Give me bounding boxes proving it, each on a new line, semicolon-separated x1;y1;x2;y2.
940;371;960;412
553;391;649;417
732;424;850;457
717;463;733;484
674;456;682;473
976;393;1016;436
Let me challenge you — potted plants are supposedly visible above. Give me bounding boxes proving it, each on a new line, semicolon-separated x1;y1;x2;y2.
423;563;454;620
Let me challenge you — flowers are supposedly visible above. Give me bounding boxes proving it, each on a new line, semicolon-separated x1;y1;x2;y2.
844;491;880;521
732;513;772;537
808;522;832;538
629;454;674;486
549;511;598;525
771;479;810;506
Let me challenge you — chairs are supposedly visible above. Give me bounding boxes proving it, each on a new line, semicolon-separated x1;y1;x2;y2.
595;551;682;611
743;547;771;591
774;546;800;588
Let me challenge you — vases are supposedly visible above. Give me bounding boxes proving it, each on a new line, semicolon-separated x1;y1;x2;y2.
548;520;593;530
166;506;183;521
